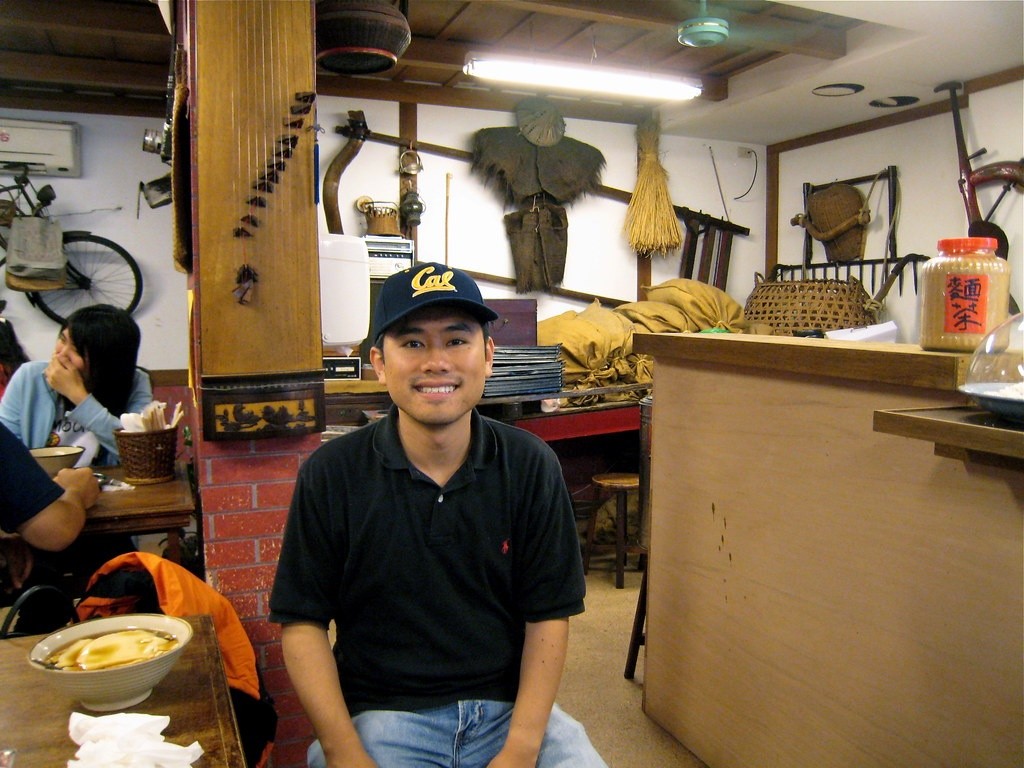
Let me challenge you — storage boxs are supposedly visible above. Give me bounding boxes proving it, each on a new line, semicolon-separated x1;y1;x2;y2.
115;428;175;485
482;300;538;348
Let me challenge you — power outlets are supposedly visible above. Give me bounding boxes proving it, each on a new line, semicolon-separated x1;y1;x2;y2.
736;146;759;160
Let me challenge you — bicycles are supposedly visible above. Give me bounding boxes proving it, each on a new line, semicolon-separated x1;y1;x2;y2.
0;162;143;326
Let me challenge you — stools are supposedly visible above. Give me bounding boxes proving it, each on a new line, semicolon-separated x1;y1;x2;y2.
582;473;648;590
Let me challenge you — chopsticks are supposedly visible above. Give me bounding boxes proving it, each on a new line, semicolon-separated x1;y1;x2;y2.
142;402;184;431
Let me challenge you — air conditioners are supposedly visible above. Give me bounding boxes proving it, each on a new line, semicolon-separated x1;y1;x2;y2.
0;118;81;180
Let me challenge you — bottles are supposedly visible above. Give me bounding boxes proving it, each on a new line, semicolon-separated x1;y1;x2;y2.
918;238;1011;352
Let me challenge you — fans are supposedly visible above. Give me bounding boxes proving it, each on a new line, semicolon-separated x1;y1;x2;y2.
653;0;799;51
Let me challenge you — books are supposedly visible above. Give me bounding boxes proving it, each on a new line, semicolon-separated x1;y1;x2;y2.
484;345;566;396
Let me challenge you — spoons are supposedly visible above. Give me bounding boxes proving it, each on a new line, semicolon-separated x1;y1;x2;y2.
121;413;144;432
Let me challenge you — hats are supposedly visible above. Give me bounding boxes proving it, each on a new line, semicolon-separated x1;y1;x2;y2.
373;262;500;348
516;97;566;149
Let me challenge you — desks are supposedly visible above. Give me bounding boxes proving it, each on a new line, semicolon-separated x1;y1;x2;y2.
0;458;197;571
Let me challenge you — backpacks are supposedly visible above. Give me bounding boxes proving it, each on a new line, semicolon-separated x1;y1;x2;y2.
66;550;279;768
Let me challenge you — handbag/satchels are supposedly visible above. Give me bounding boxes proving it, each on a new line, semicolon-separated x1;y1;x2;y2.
5;216;67;292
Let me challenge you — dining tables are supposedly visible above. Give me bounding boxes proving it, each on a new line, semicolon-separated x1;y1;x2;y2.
0;615;256;765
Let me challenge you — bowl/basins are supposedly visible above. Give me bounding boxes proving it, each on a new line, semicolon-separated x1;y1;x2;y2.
29;446;86;480
27;614;193;711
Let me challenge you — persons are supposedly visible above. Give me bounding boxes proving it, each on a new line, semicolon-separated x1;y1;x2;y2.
268;262;609;768
0;300;153;634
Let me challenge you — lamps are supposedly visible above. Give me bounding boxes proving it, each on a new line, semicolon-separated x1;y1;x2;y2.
462;54;708;103
399;190;426;227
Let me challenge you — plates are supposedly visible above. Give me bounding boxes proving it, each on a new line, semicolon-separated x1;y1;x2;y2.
958;383;1024;420
